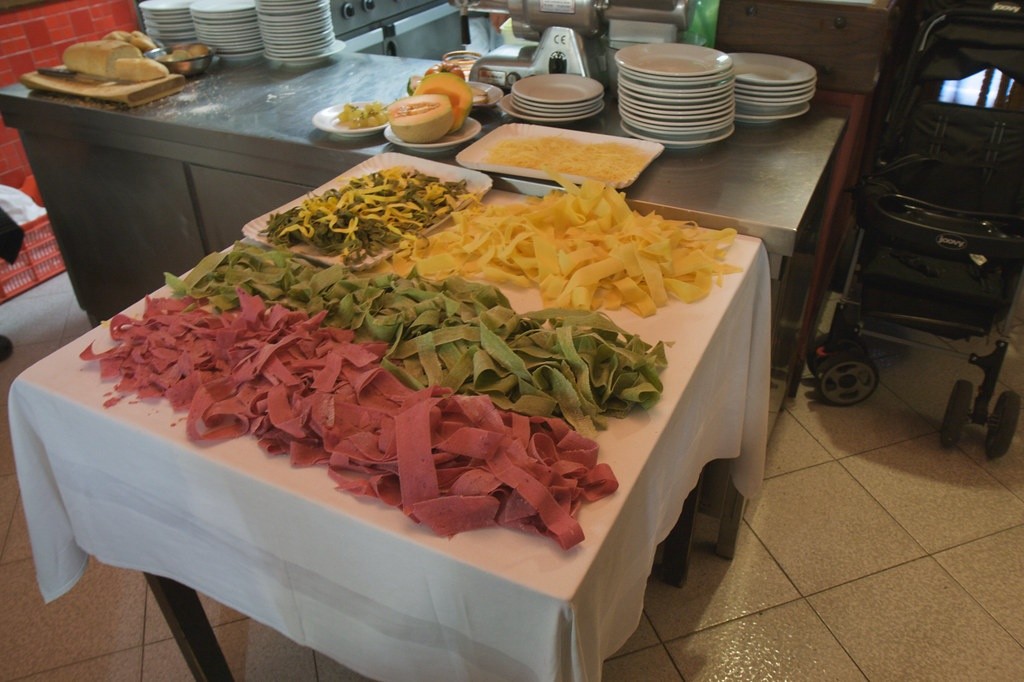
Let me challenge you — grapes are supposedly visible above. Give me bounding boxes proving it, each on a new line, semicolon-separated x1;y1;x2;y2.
338;100;389;129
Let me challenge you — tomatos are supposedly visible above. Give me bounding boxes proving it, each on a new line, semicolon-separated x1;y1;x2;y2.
425;63;465;81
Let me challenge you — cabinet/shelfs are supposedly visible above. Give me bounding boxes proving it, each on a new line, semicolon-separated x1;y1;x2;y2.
0;52;848;561
712;1;901;256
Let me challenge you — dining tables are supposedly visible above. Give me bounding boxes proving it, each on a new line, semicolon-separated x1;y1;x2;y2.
7;168;774;682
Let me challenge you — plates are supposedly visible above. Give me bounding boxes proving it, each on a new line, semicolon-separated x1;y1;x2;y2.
467;81;503;111
384;117;481;153
313;103;390;138
139;0;345;66
241;152;492;270
614;44;818;149
499;73;604;124
455;124;664;189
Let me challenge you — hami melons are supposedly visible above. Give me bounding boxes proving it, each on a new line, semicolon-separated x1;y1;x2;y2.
387;72;474;144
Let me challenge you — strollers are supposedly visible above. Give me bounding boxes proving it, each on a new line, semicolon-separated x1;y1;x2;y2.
804;7;1024;459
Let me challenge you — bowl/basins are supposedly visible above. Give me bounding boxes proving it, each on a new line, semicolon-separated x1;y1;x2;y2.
144;45;218;76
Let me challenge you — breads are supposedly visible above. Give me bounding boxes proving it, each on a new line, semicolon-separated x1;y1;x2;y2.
62;31;170;81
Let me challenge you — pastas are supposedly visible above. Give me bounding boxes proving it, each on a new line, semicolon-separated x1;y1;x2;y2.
487;137;646;184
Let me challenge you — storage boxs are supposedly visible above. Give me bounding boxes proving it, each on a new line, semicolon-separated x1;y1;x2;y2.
0;211;67;305
